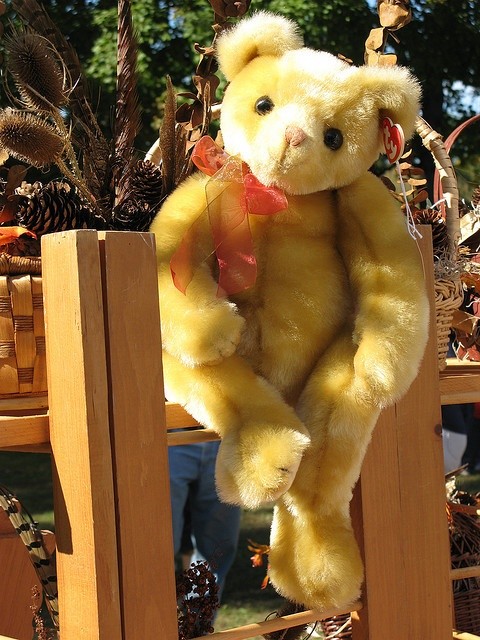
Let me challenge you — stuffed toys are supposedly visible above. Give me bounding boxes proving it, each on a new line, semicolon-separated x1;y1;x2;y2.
149;11;431;613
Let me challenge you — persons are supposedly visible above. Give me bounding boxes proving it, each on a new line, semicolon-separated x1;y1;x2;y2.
170;428;241;621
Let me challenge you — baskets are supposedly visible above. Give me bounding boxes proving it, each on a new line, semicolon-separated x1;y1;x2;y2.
1;252;49;416
417;123;465;371
